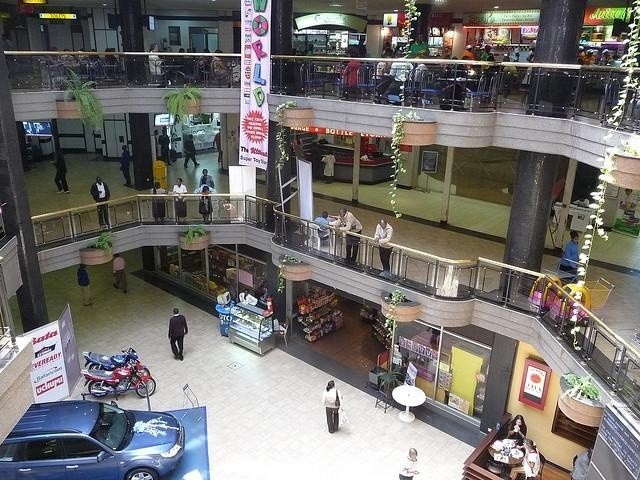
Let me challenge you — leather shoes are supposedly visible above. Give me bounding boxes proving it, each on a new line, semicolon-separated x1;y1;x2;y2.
180;356;183;360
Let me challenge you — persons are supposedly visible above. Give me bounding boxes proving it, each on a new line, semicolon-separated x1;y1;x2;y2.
329;208;363;262
77;264;94;307
51;147;72;195
322;152;337;182
504;415;527;444
372;34;536;106
374;219;394;279
313;211;331;239
511;439;541;480
323;381;342;434
149;42;229;84
90;127;220;229
50;46;123;78
561;229;581;282
572;196;589;207
619;189;636;210
399;447;421;480
111;254;128;293
343;39;367;86
168;308;188;360
577;48;624;105
6;32;17;78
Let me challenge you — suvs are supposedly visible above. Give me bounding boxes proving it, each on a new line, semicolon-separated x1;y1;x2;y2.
0;400;185;480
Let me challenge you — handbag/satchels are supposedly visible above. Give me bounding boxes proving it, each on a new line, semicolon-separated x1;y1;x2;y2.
335;398;340;406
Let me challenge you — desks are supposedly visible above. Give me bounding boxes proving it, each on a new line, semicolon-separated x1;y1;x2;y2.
392;385;428;422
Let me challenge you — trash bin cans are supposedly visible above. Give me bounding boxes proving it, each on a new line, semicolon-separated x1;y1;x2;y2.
152;160;168;189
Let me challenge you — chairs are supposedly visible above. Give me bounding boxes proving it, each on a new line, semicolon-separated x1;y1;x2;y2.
307;222;332;254
38;48;243;89
273;321;290;347
538;451;546;480
296;60;510;112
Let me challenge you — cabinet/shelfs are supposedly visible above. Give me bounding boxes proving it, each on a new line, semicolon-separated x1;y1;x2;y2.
228;305;275;357
299;300;339;343
291;132;355;160
164;244;267;301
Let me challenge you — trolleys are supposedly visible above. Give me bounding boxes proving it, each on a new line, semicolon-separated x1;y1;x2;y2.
552;277;616;325
622;327;640;370
529;258;586;311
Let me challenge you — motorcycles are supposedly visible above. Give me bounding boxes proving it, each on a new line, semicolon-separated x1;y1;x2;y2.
82;347;139;368
80;356;156;398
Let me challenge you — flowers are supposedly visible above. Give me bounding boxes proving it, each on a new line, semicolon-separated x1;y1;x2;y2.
381;287;408;341
560;371;601;403
274;100;297;169
387;110;424;220
277;253;302;294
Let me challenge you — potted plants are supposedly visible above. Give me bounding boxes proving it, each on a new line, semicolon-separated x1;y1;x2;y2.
175;224;211;250
160;83;203;124
604;133;639;192
79;230;116;266
54;68;105;132
